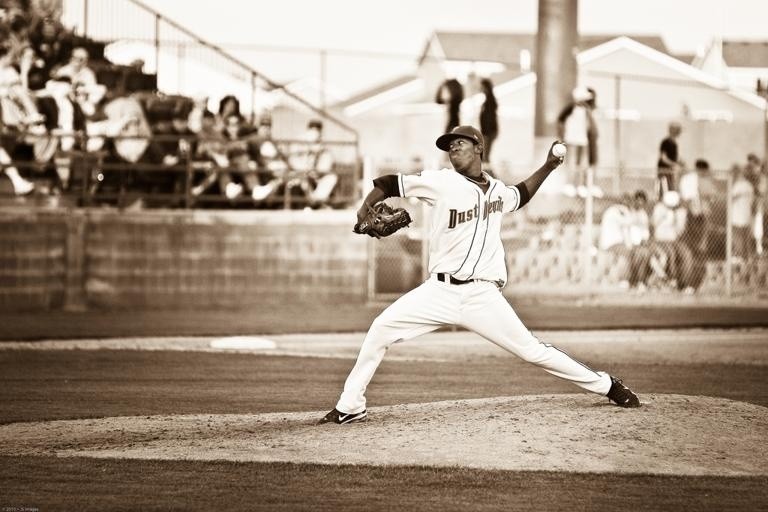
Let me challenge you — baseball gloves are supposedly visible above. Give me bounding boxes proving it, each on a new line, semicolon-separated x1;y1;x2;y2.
352;204;412;238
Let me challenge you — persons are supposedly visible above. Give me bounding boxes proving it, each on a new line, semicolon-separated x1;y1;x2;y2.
319;125;643;409
479;78;498;179
558;86;767;291
437;80;464;134
0;0;340;210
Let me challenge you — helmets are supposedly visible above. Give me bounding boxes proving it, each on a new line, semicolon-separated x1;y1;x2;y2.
436;125;485;151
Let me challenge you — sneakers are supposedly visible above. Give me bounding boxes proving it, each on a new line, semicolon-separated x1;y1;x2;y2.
316;408;367;426
606;377;641;408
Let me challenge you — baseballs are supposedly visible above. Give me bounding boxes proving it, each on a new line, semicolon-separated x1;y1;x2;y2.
553;144;566;157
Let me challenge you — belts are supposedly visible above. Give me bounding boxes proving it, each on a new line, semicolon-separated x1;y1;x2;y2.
436;272;474;285
